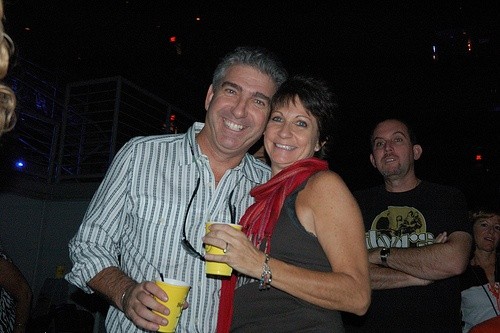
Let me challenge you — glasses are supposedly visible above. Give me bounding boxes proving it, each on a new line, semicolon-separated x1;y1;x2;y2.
181;178;244;264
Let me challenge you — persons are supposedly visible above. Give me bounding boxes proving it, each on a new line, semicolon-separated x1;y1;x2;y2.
340;114;474;332
0;244;34;333
65;42;288;333
200;70;372;333
458;206;500;333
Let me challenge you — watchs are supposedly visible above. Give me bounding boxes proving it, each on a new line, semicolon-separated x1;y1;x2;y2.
380;247;391;267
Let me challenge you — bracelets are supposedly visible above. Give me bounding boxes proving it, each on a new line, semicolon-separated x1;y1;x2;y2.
14;322;27;327
258;254;273;290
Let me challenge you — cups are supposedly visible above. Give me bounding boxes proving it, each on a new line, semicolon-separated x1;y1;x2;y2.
151;278;190;333
205;222;243;277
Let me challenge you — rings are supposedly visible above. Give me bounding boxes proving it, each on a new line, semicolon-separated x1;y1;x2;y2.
223;242;228;254
